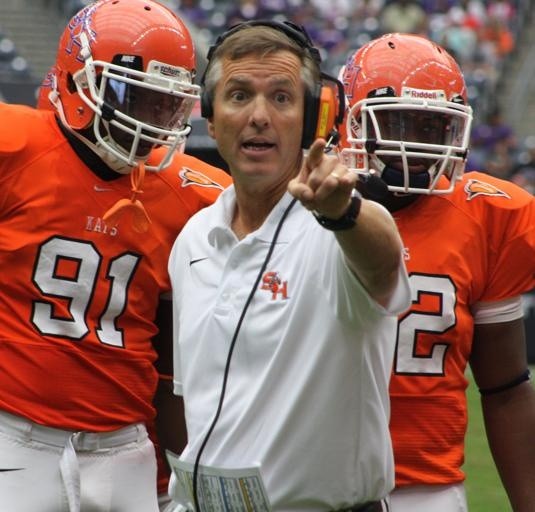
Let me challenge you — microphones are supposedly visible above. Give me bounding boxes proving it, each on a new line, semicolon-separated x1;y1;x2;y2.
323;129;339;153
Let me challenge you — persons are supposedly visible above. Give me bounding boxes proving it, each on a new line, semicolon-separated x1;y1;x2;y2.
334;30;535;512
164;19;416;511
1;0;236;511
184;0;535;204
0;34;41;108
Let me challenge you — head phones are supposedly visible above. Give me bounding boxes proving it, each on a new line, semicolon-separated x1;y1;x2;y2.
200;20;343;149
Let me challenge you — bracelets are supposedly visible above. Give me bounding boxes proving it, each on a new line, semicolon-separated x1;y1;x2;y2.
309;188;365;232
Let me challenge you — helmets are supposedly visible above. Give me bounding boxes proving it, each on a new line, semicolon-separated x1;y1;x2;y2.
334;33;473;211
38;0;200;180
